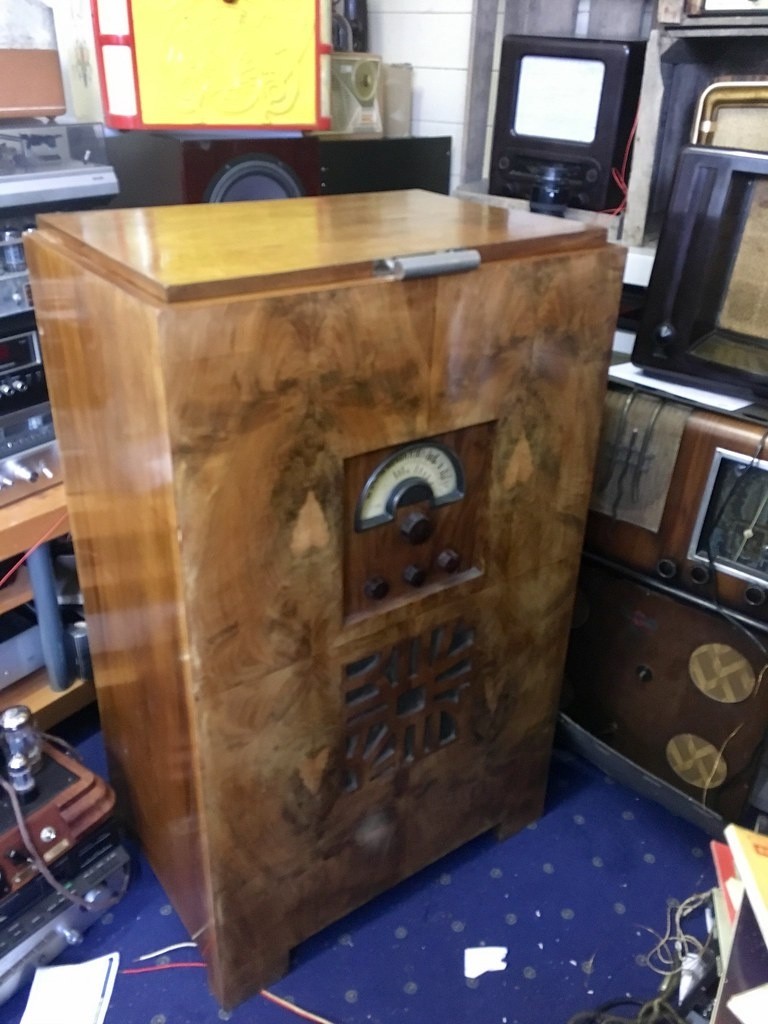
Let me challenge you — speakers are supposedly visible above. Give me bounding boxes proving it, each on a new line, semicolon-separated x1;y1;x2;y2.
106;130;451;206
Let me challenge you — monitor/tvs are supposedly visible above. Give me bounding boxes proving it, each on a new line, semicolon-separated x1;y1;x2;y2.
515;54;605;144
